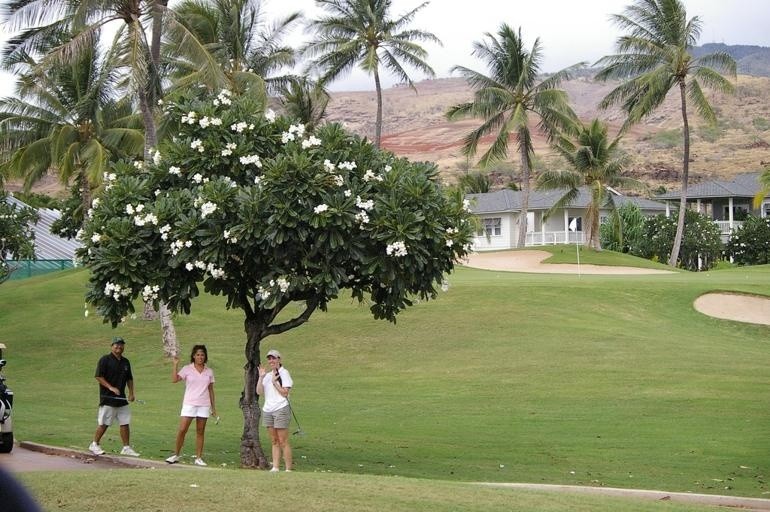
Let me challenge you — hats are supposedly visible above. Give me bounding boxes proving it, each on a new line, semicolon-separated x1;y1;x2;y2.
266;349;280;358
111;336;125;346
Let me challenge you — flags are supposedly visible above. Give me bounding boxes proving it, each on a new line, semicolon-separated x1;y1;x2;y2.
568;217;576;232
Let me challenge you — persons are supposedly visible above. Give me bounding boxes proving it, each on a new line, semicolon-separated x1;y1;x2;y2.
256;349;293;472
88;337;141;457
166;345;216;466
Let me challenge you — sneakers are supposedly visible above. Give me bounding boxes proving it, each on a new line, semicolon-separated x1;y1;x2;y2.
195;458;207;466
89;442;106;454
120;448;141;457
269;467;279;472
165;455;179;464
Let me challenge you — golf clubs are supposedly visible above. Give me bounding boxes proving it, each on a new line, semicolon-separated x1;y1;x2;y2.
209;410;220;425
275;368;301;435
101;395;146;405
0;343;6;372
